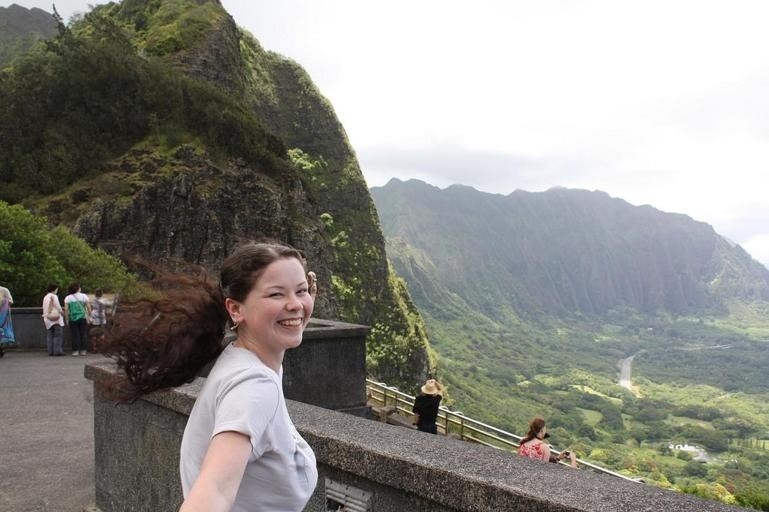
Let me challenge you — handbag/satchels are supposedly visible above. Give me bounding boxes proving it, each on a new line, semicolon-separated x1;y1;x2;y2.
85;311;95;325
46;310;61;321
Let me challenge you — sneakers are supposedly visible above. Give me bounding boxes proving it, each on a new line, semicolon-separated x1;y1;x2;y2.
68;347;110;358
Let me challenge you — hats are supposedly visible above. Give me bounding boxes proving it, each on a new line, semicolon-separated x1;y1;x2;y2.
420;378;440;395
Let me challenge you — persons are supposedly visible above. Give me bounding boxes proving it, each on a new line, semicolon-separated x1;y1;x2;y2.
544;433;578;470
412;379;443;435
296;247;318;300
42;281;68;358
62;282;93;357
89;287;117;354
110;242;320;510
0;284;14;362
518;417;551;464
